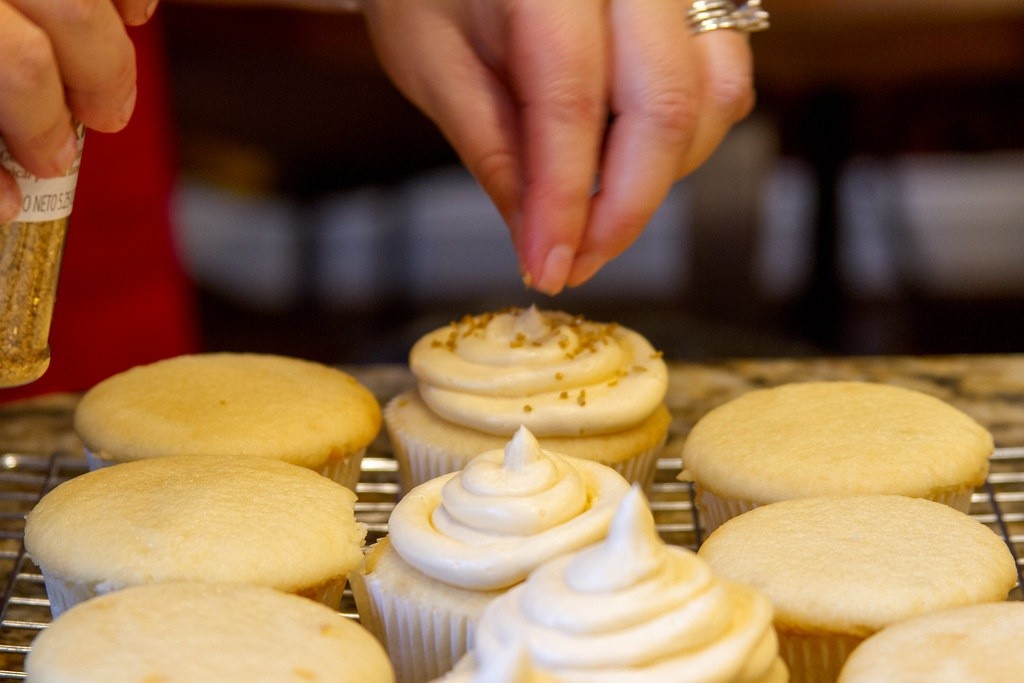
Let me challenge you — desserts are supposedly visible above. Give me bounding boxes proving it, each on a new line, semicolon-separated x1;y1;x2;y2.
24;304;1024;683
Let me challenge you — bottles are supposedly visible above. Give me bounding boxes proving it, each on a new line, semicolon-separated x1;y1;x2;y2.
0;115;84;388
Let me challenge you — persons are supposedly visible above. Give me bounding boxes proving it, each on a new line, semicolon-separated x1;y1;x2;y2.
0;0;756;406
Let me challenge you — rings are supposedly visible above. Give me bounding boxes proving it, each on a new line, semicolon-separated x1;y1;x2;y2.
683;0;771;39
